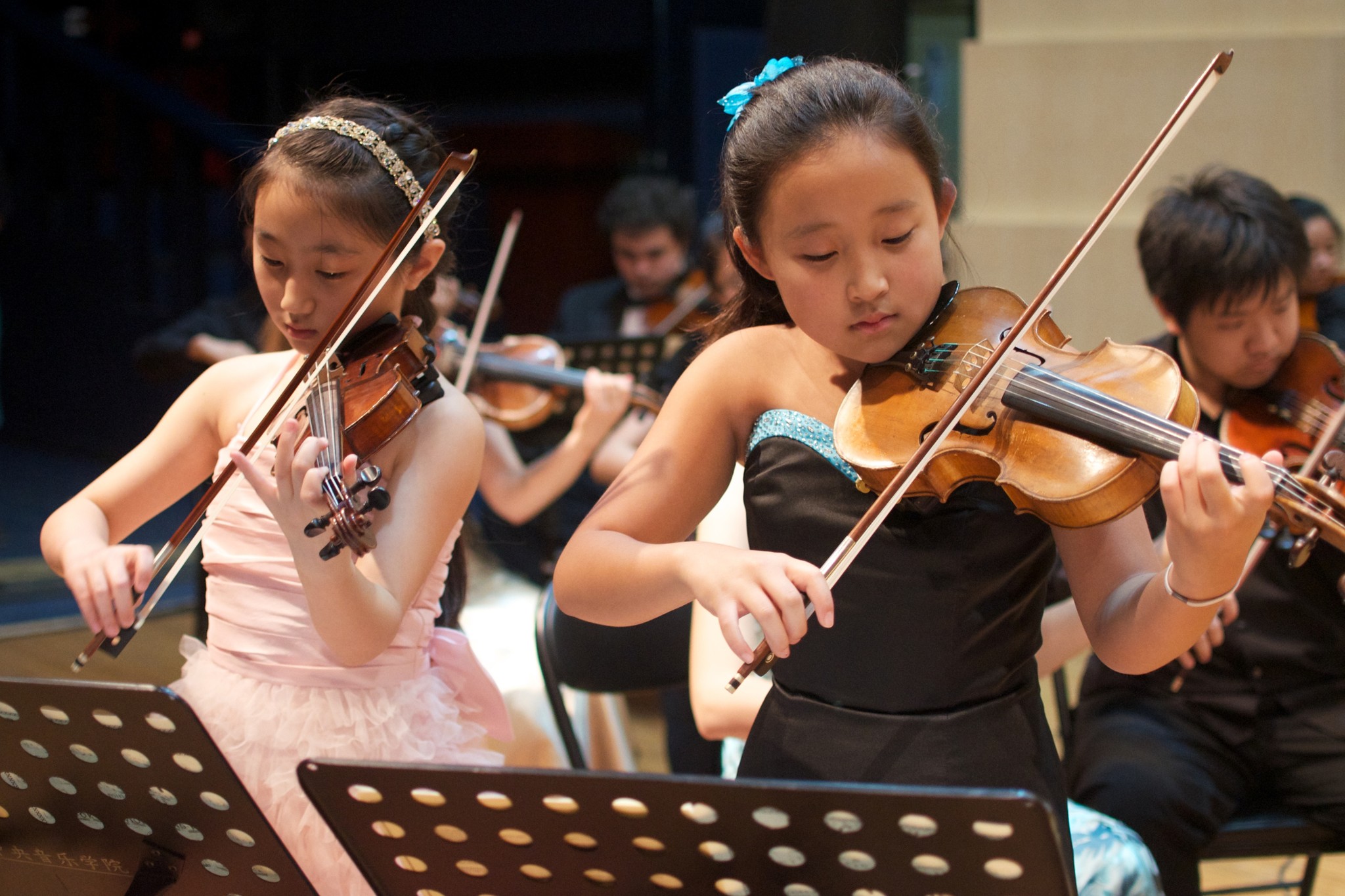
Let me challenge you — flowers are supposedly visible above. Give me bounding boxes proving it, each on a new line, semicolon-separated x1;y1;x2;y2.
716;54;807;132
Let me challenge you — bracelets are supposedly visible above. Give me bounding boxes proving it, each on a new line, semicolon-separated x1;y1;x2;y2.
1165;560;1242;607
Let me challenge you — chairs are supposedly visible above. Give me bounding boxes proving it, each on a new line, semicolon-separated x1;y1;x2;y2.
527;573;722;787
1050;643;1333;896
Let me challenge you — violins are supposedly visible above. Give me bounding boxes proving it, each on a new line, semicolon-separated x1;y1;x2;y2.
1219;332;1345;534
622;266;726;334
270;317;445;562
832;286;1344;570
429;318;664;430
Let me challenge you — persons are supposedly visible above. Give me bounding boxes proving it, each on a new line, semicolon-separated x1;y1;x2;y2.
37;91;507;896
158;149;1345;896
550;54;1287;896
